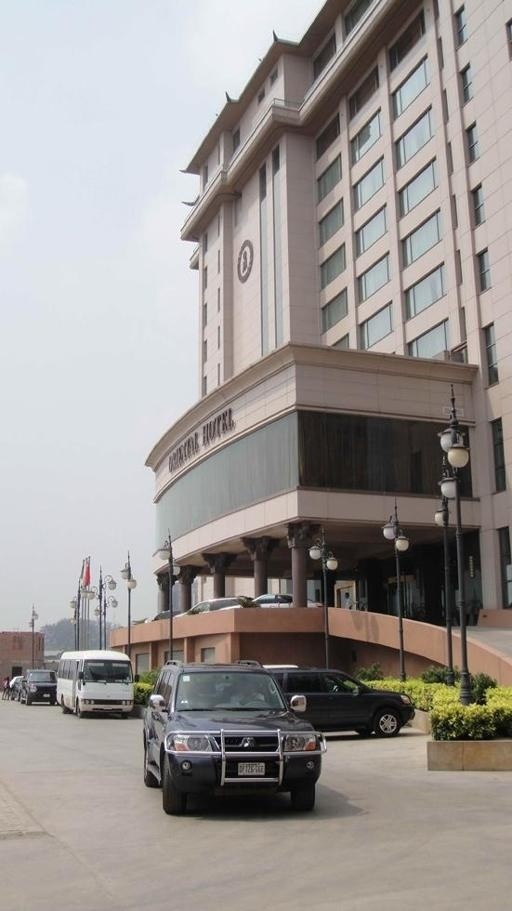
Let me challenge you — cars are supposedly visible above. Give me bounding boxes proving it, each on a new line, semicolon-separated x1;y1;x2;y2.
145;593;324;623
9;676;24;702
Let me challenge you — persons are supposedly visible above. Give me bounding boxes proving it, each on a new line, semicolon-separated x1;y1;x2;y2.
2;676;10;700
231;680;265;705
345;592;354;610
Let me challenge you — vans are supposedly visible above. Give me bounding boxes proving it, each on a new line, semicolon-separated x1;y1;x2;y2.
54;648;141;719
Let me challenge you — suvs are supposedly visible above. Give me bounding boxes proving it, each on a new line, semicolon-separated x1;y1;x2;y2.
140;658;325;827
20;670;57;706
251;663;416;739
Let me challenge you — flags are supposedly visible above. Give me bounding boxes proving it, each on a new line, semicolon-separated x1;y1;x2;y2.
80;558;90;587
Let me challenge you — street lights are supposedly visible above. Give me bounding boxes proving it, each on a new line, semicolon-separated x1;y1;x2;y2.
70;556;119;655
121;550;137;660
310;528;339;669
435;380;479;704
380;493;409;681
28;603;39;670
157;529;182;666
